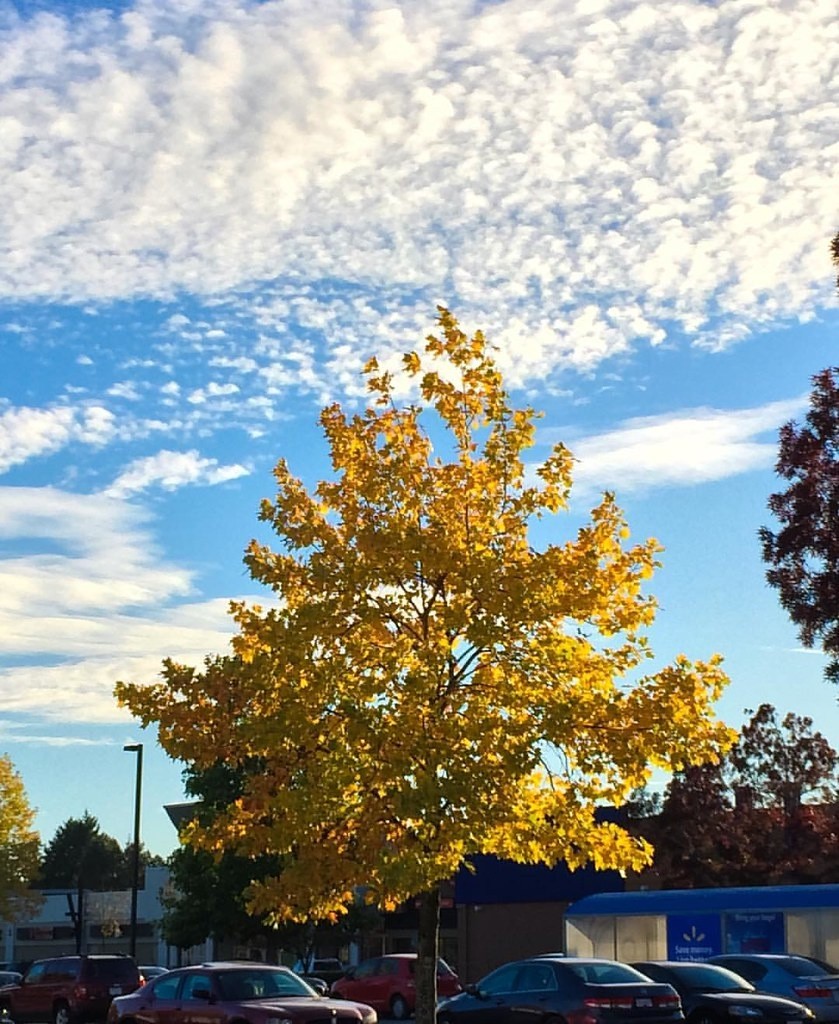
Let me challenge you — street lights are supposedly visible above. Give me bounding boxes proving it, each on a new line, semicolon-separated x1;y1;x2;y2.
123;744;144;957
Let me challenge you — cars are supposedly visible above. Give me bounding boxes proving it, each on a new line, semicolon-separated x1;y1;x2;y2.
330;953;462;1021
108;961;378;1024
624;960;817;1024
706;953;839;1024
435;953;686;1024
0;953;346;1024
